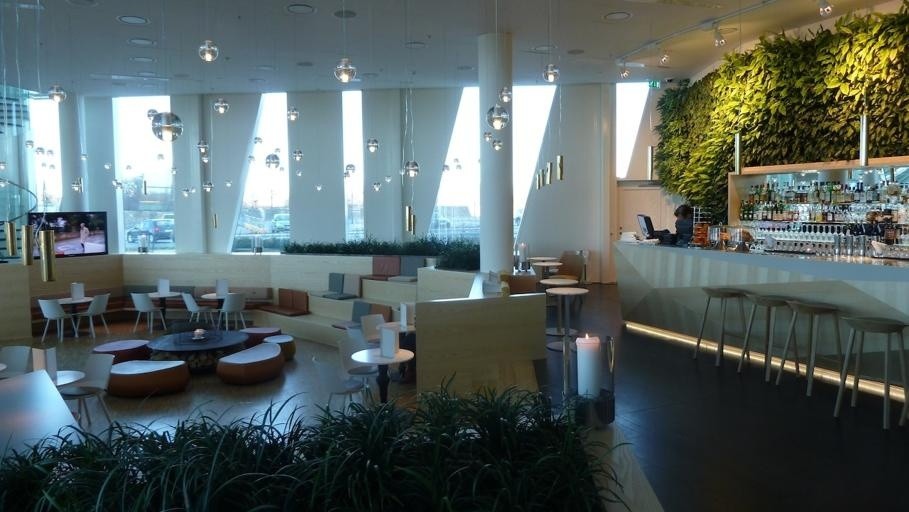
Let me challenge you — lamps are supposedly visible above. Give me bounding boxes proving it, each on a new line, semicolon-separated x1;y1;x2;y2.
713;22;727;48
819;0;832;17
0;0;564;281
620;58;631;79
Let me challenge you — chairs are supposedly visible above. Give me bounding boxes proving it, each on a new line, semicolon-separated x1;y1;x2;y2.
37;281;415;427
0;346;31;380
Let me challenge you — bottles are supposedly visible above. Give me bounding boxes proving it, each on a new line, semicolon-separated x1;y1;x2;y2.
859;182;909;259
739;180;803;253
802;181;859;255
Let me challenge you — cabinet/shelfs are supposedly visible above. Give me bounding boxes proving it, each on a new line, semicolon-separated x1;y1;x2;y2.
728;156;909;258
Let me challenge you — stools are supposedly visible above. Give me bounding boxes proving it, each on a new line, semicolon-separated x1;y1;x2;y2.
692;287;751;367
737;292;800;383
833;317;909;430
775;299;850;398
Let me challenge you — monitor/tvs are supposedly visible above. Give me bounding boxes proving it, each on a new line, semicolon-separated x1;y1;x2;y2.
28;211;108;260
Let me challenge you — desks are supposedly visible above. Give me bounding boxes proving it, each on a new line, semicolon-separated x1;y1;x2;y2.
0;370;90;482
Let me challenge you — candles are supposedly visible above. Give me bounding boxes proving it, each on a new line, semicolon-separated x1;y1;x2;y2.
575;336;601;398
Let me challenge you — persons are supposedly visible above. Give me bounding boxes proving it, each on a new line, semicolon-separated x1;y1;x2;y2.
79;222;91;254
674;204;705;234
